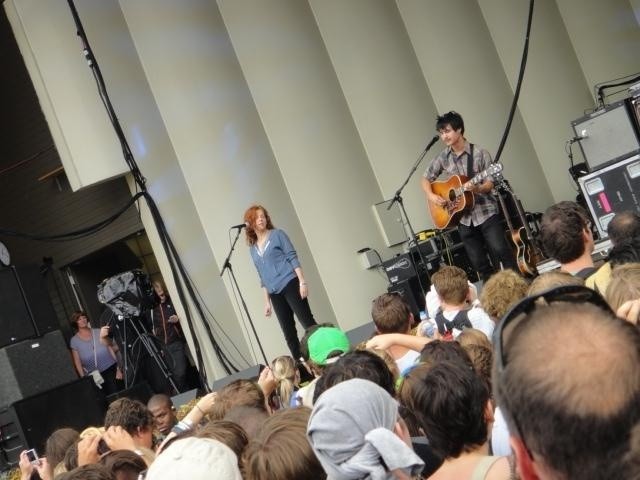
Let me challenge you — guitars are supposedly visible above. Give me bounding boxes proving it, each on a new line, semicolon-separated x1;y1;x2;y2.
427;162;503;230
503;180;549;279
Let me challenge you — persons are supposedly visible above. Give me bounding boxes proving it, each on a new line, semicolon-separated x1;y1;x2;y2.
18;205;639;480
421;112;518;283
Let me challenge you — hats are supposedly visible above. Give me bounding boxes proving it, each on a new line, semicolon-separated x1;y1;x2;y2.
307;327;351;366
143;437;240;480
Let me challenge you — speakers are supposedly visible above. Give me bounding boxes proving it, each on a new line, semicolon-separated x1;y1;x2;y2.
0;328;81;410
344;321;377;347
170;388;208;411
0;263;62;349
0;375;111;475
212;364;266;393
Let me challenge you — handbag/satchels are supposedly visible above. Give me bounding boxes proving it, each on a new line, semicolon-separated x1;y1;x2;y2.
89;370;105;390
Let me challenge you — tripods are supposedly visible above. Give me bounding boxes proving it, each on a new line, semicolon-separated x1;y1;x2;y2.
105;311;181;395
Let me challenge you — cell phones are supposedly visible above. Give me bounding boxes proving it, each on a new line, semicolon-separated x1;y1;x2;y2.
26;448;41;466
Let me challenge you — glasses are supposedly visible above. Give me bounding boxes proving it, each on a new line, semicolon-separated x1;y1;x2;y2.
492;284;616;461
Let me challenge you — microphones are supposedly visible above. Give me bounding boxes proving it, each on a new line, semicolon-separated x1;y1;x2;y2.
232;222;250;229
425;135;440;152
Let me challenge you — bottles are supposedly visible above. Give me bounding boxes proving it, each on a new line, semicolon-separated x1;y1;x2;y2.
420;311;434;337
